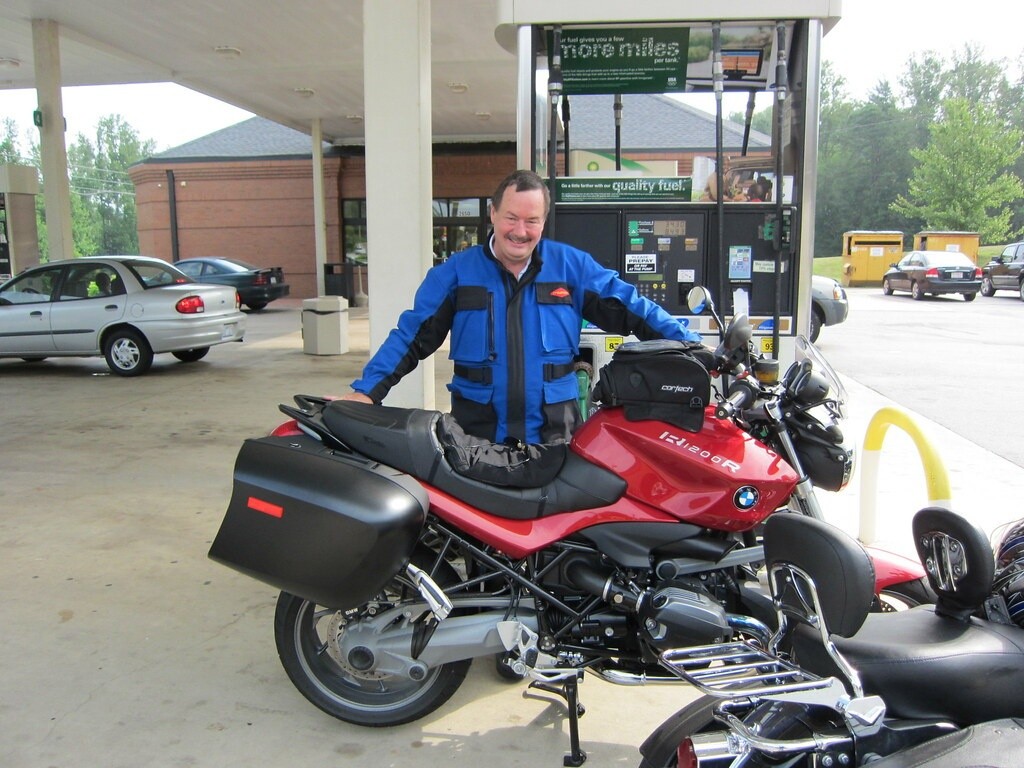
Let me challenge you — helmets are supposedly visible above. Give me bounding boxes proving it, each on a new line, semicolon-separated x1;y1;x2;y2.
992;519;1024;627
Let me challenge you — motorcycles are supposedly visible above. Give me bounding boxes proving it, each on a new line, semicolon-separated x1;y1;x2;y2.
637;505;1024;768
209;278;936;768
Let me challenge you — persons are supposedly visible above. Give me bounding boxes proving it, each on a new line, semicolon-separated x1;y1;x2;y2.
698;155;735;202
95;272;122;297
322;169;721;682
747;176;772;202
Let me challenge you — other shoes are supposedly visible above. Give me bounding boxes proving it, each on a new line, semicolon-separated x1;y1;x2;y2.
495;649;525;680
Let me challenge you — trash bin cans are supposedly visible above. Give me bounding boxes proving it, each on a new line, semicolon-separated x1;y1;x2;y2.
323;263;356;307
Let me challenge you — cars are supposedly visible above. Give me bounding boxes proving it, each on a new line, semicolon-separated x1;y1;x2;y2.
882;249;983;302
0;255;250;379
809;275;849;343
139;256;290;311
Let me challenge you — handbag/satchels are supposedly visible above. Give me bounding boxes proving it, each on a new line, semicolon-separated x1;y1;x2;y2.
594;338;711;433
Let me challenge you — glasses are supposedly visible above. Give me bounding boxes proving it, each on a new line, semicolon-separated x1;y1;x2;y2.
468;436;530;468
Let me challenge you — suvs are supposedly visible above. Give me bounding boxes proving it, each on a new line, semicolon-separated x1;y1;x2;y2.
980;242;1024;302
